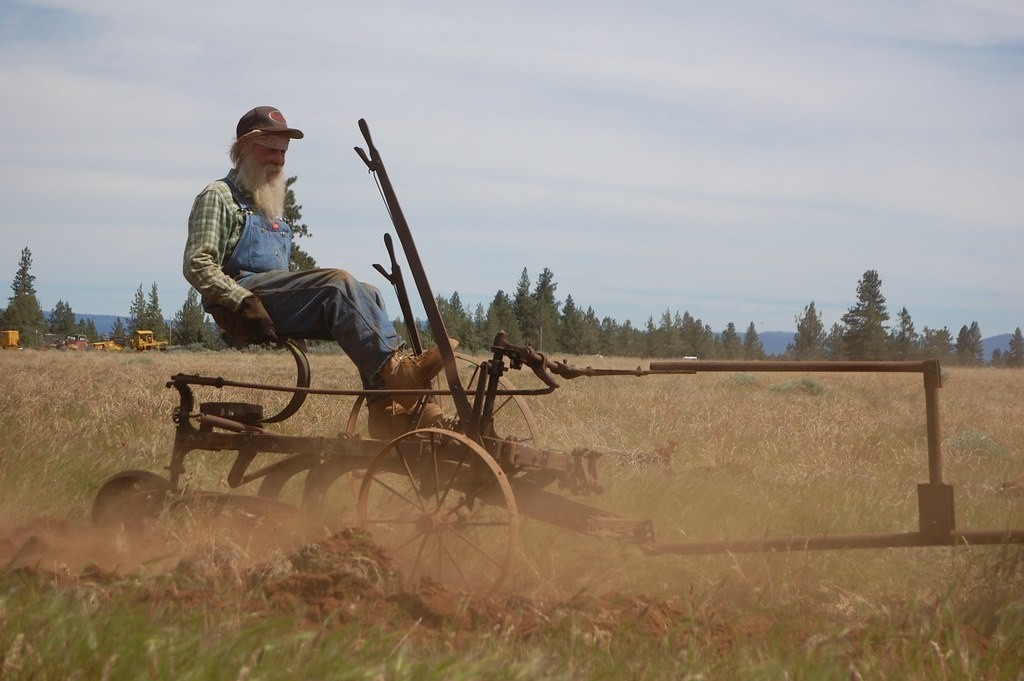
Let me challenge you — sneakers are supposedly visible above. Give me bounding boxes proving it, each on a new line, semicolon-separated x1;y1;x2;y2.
368;396;444;438
380;338;459;410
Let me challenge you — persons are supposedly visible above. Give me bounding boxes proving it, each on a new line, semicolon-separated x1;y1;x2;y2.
182;105;460;438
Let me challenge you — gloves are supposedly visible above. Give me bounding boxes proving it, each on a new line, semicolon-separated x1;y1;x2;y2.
240;295;273;344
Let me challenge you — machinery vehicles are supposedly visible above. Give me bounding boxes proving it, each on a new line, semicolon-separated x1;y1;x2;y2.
130;331;168;353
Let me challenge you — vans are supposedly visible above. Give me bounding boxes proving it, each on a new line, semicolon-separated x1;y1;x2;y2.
0;331;22;349
66;334;86;350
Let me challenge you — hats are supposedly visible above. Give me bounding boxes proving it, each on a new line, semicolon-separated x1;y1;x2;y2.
236;106;304;140
